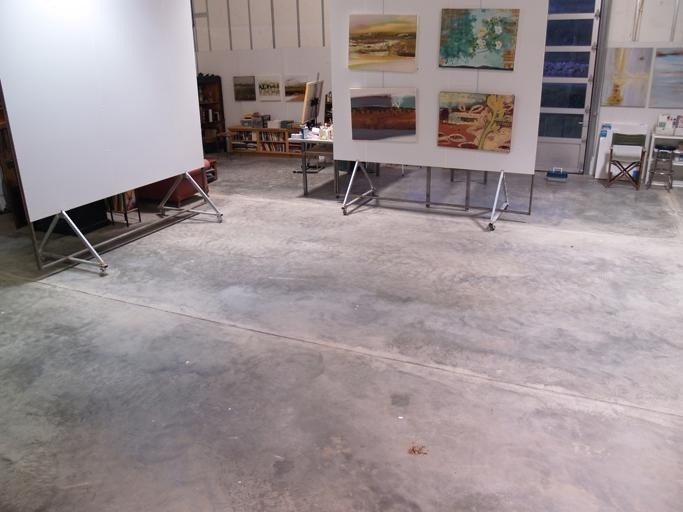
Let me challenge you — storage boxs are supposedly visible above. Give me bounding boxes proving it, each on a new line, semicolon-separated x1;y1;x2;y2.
546;166;569;183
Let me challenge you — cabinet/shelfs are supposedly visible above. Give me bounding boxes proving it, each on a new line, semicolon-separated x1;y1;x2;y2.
645;133;683;189
227;123;304;159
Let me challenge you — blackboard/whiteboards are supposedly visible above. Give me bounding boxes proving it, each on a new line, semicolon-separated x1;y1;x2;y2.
1;0;204;222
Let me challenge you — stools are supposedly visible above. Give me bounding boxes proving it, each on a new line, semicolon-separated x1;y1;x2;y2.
646;141;675;190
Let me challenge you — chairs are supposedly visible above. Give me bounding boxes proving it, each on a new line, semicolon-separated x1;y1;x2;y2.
607;125;647;189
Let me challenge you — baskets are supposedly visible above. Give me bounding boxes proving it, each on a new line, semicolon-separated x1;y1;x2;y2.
547;167;568;182
